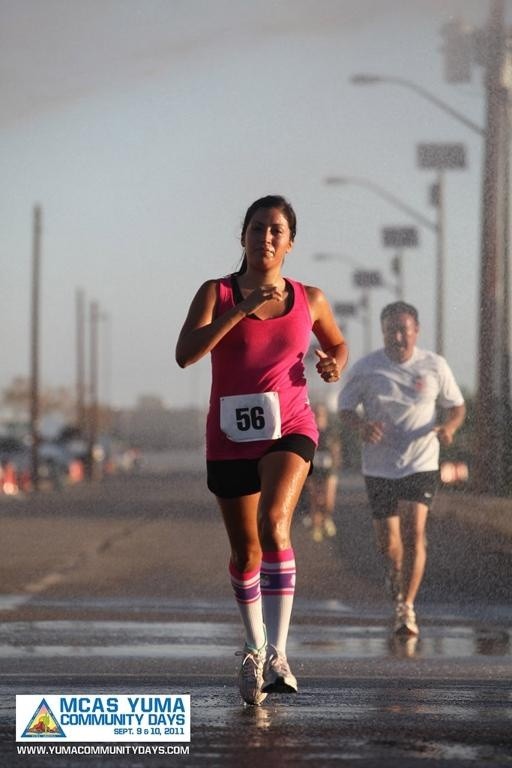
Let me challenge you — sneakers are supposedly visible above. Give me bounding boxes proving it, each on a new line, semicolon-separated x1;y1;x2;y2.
235;624;297;706
310;515;336;543
393;591;419;641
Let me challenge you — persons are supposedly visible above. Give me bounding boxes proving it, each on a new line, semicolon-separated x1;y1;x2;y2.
339;302;468;635
175;196;349;705
311;403;342;542
